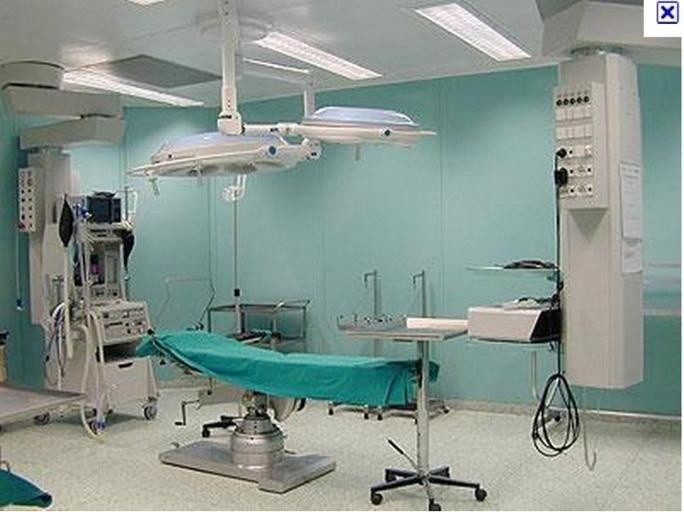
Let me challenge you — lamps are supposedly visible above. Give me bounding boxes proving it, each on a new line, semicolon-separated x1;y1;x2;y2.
128;1;312;180
247;91;438;147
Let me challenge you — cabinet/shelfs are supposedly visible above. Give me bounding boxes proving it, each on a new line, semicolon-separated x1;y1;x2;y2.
208;299;310;395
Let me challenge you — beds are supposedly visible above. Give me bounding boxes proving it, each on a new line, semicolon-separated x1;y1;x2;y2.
134;330;439;493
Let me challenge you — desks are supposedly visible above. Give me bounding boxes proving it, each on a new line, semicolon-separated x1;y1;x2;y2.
348;316;486;511
